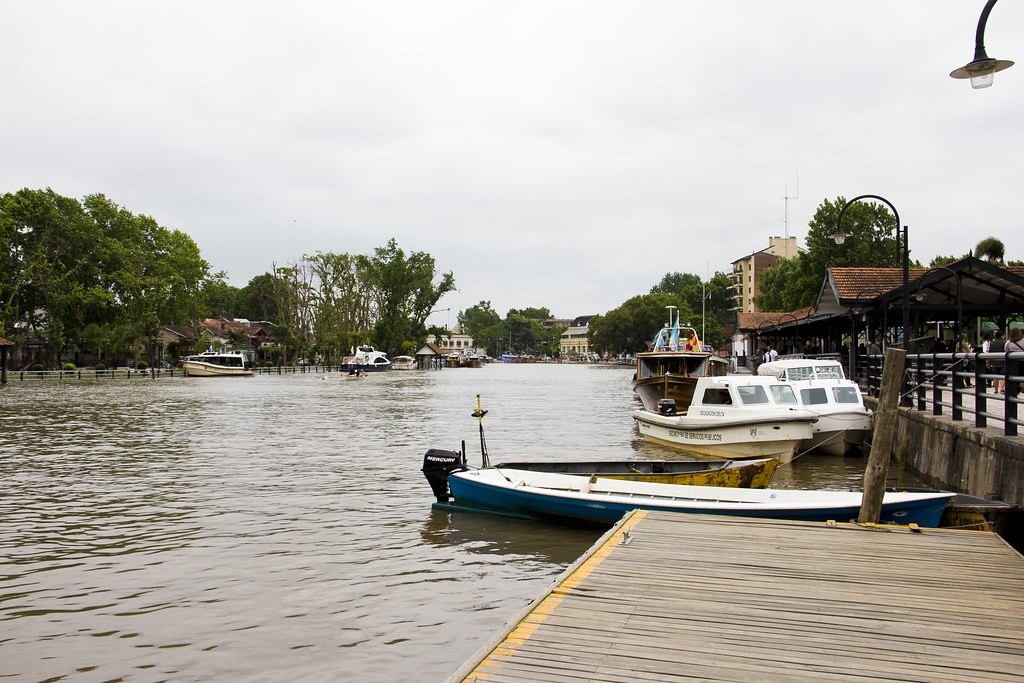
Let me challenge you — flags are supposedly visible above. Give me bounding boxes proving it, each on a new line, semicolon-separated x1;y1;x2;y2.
690;335;699;352
669;319;680;351
653;330;665;352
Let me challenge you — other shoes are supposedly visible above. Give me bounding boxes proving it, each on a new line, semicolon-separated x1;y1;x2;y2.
967;384;973;388
1000;390;1005;395
1019;384;1024;393
994;390;998;393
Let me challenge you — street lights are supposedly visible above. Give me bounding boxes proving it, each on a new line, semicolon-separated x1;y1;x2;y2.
832;196;913;407
703;285;713;356
45;336;49;375
106;338;111;374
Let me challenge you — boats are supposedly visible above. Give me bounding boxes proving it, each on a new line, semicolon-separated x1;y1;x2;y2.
339;346;392;372
180;351;254;376
342;375;367;380
441;355;495;368
883;484;1020;544
630;373;819;468
389;356;418;370
501;355;537;363
635;306;730;414
419;393;961;533
756;359;876;455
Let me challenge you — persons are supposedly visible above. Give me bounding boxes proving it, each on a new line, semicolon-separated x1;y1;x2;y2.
804;341;814;359
954;327;1024;395
686;334;694;351
885;340;890;350
751;345;779;375
865;341;882;374
348;369;363;377
